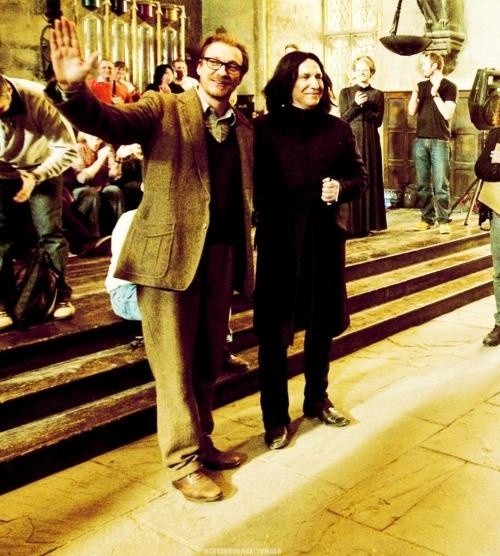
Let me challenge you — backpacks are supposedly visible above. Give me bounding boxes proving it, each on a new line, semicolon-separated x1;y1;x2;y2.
3;248;58;326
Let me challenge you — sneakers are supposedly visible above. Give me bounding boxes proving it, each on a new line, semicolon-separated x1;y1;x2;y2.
52;298;74;320
1;307;12;330
437;221;451;235
484;326;500;346
410;218;434;231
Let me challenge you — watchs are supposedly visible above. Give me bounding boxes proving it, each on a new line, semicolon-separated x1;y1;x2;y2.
432;93;440;99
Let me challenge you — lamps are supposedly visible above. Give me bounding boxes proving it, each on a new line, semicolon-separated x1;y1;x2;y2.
378;1;433;56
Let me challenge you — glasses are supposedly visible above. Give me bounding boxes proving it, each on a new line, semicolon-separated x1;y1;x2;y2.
199;55;245;76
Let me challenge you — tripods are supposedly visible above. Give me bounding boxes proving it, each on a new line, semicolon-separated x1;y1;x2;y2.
449;178;490;227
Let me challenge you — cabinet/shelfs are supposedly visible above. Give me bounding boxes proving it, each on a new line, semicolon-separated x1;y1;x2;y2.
382;90;490;209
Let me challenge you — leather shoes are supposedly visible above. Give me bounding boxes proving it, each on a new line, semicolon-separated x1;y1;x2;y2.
173;467;223;502
263;420;289;451
195;444;246;469
303;405;351;427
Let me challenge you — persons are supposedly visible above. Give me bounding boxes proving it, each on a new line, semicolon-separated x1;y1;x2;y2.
338;54;387;239
67;55;200;241
0;73;81;327
47;14;259;506
475;92;500;347
250;48;368;452
407;52;458;234
106;184;250;371
